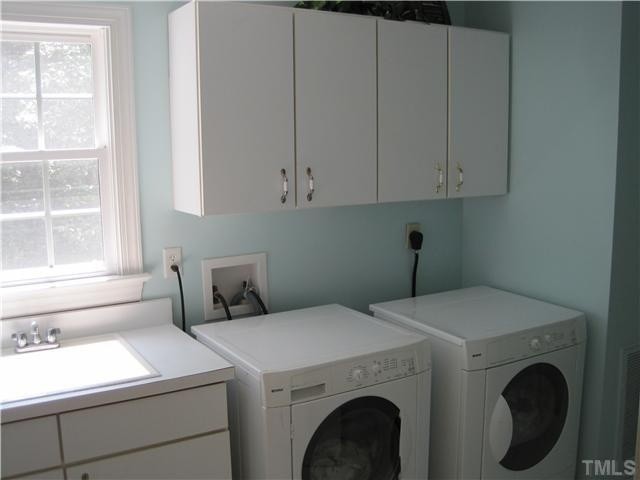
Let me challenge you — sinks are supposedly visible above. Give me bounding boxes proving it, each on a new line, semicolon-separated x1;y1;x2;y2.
0;332;163;405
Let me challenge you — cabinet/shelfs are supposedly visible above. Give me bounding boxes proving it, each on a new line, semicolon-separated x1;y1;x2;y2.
1;415;67;479
58;380;232;479
378;16;513;209
163;0;378;220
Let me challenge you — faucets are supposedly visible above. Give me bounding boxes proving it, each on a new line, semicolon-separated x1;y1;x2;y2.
30;322;42;344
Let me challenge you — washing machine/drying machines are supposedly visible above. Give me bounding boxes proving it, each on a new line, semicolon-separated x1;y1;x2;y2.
368;285;589;480
188;303;434;480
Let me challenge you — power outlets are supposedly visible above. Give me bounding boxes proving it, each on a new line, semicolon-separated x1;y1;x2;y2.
405;221;422;251
163;245;185;282
